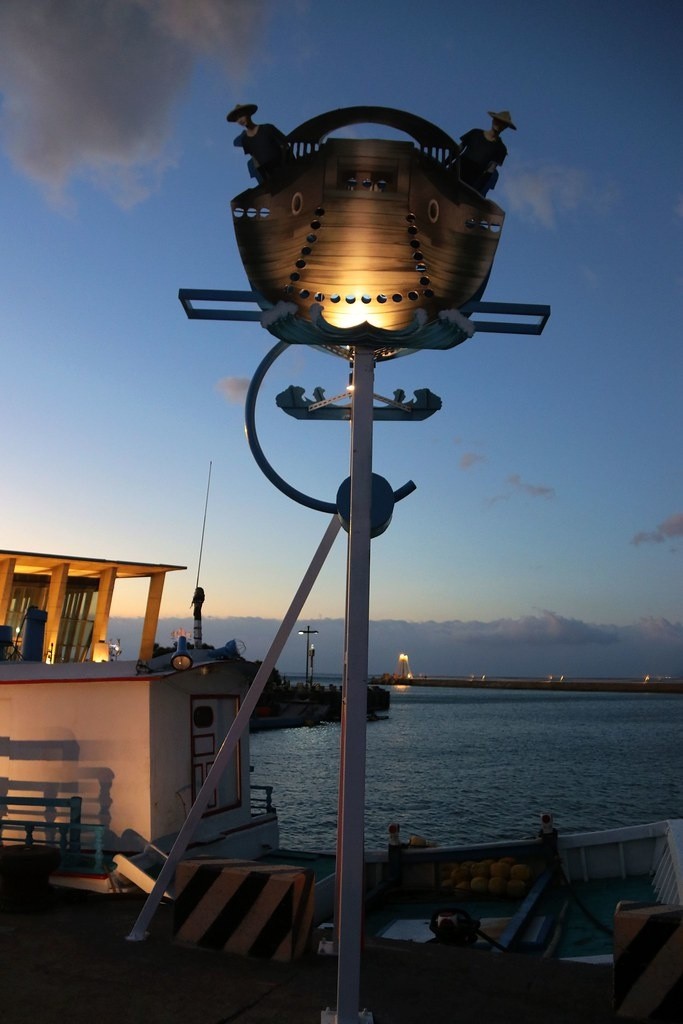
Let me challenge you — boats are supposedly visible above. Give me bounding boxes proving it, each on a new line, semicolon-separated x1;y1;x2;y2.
296;624;392;715
151;460;328;728
